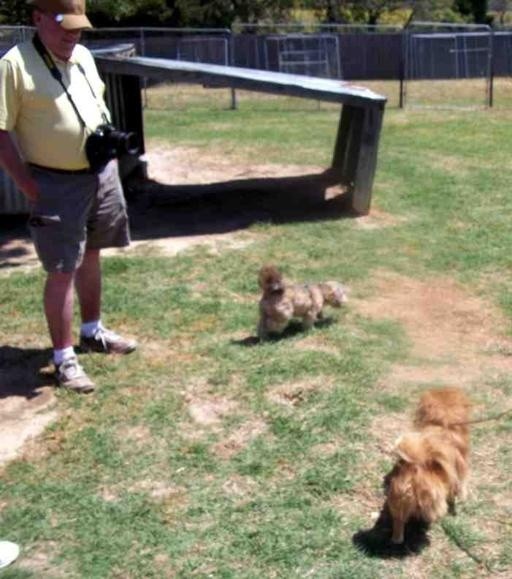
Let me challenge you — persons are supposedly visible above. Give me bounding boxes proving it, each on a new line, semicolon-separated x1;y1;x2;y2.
0;0;137;393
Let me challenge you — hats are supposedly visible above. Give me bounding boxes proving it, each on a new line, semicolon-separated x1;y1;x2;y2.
36;0;93;32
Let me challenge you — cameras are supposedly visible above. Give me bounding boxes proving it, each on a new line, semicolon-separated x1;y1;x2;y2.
85;124;139;174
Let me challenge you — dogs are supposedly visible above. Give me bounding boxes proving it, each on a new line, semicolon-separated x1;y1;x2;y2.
259;260;351;346
386;386;475;544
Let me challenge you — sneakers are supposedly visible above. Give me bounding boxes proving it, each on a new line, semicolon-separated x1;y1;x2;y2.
55;357;95;393
80;328;138;354
